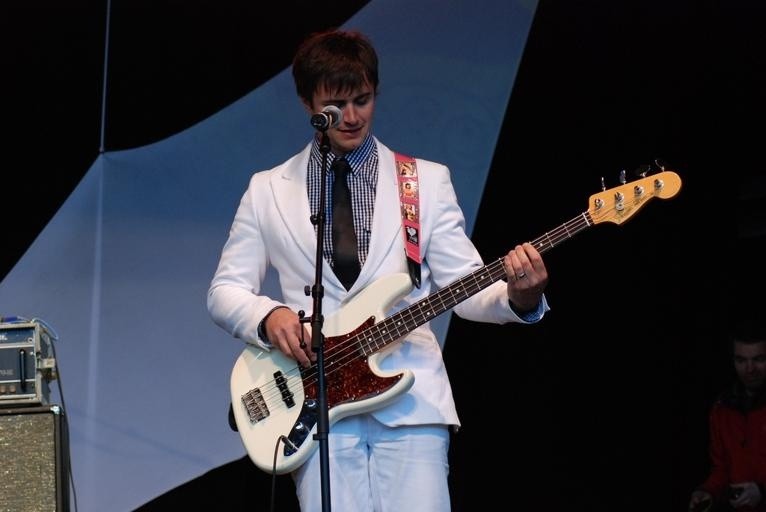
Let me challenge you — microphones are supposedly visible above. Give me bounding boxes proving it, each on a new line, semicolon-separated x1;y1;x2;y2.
310;105;343;129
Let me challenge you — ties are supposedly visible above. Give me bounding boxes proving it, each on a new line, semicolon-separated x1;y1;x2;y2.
327;155;362;292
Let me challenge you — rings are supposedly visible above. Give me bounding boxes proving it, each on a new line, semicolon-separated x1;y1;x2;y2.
518;272;526;279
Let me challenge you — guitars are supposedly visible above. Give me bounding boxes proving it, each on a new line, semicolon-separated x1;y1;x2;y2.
231;157;683;473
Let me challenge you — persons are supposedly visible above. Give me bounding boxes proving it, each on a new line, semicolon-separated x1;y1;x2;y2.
206;30;552;512
688;319;766;512
